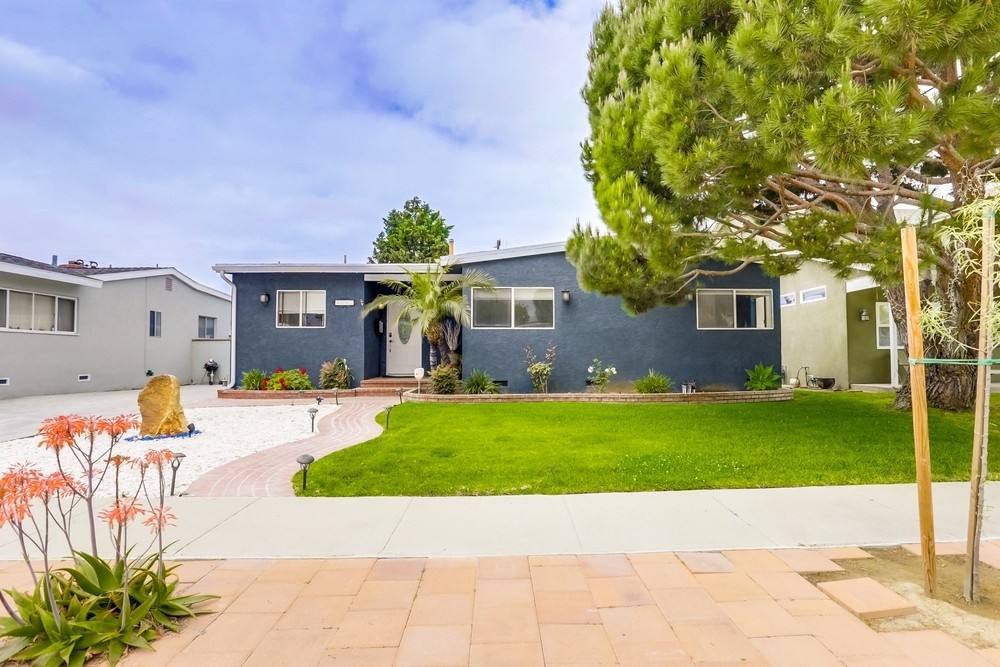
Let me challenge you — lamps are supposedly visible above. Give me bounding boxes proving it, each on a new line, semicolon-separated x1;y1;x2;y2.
259;288;270;304
562;288;570;302
168;453;186;495
308;408;318;431
861;310;870;320
332;388;339;404
297;455;314;490
316;396;322;405
187;423;195;438
383;405;393;429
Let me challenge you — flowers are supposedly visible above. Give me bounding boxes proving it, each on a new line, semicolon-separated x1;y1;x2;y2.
262;368;316;391
